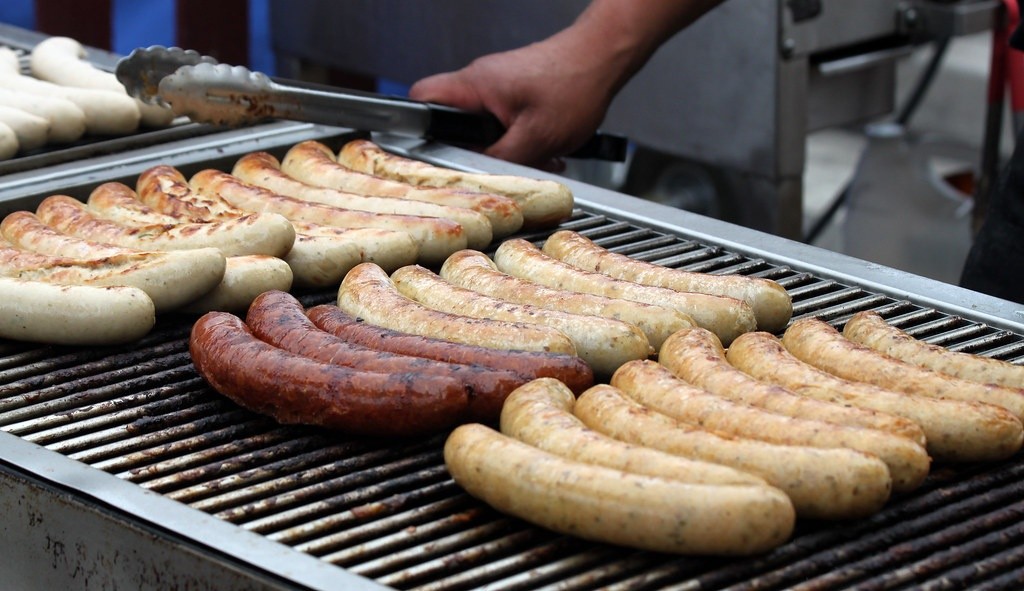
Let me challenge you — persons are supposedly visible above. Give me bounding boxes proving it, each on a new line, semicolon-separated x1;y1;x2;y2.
412;1;1023;307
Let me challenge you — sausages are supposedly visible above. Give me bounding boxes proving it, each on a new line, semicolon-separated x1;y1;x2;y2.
0;31;1024;568
